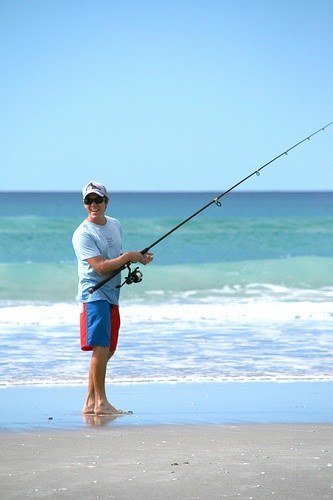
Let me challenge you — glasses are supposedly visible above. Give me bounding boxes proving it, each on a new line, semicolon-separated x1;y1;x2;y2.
84;196;106;205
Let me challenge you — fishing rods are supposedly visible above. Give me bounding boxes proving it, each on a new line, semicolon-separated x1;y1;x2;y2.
87;122;333;294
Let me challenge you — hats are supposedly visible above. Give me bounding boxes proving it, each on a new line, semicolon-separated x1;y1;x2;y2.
82;181;109;202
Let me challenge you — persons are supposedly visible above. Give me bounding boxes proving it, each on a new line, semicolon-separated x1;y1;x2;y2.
72;183;153;415
